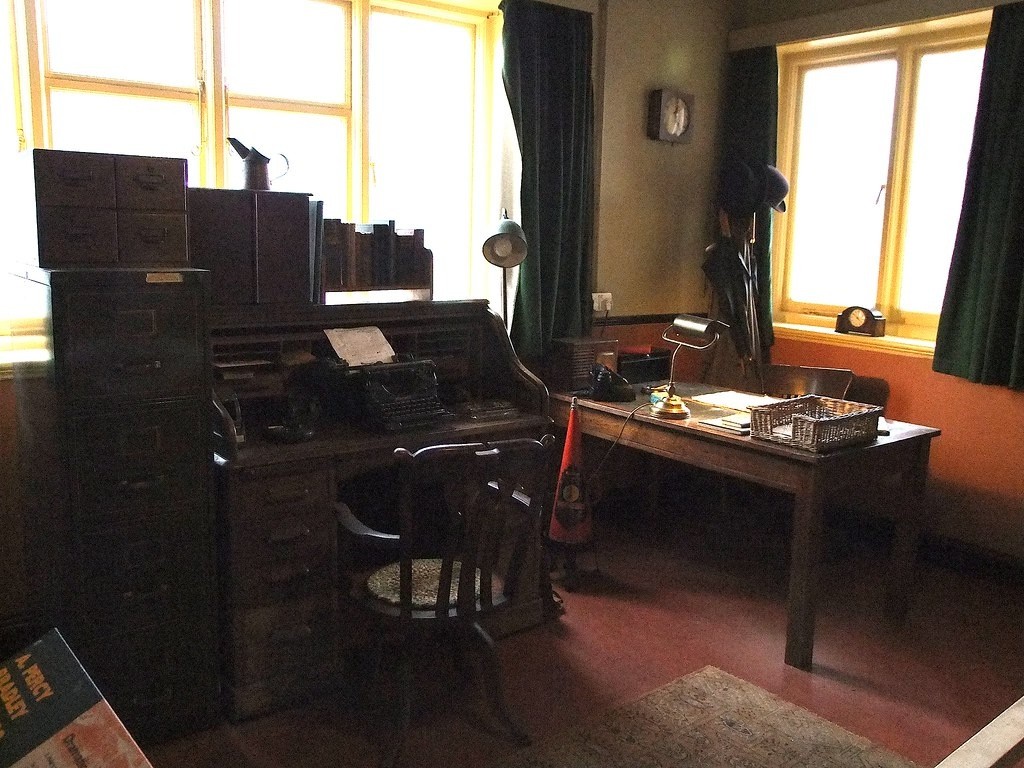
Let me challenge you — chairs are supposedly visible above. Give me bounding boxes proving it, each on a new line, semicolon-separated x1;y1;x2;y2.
751;364;853;399
329;434;553;767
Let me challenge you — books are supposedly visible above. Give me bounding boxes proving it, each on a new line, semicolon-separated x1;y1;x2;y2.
324;217;427;287
698;411;751;436
877;416;890;436
0;628;153;768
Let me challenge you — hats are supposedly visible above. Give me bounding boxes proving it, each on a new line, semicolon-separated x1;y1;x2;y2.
764;164;789;213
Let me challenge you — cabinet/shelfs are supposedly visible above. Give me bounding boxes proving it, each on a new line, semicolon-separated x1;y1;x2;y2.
43;268;219;748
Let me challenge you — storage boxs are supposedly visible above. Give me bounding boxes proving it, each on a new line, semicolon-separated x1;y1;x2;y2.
33;148;313;306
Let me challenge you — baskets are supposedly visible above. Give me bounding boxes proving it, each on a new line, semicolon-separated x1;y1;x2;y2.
746;393;884;453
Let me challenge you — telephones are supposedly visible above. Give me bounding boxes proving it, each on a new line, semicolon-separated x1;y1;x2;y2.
588;363;636;403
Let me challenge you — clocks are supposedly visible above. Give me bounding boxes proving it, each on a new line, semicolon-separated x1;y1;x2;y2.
647;89;694;145
834;306;885;337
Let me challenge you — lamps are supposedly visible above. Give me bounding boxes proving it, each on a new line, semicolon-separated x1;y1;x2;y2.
649;314;730;420
482;208;527;330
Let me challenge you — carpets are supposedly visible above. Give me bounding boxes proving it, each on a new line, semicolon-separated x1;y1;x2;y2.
496;665;919;768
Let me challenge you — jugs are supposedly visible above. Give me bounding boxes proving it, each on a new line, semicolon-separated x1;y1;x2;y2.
227;137;289;190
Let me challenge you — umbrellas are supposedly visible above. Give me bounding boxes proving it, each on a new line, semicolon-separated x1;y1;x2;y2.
701;205;763;380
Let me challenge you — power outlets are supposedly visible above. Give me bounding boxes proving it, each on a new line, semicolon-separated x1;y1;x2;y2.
592;293;611;312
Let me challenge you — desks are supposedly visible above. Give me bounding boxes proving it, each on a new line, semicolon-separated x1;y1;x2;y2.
548;378;942;671
202;297;554;726
